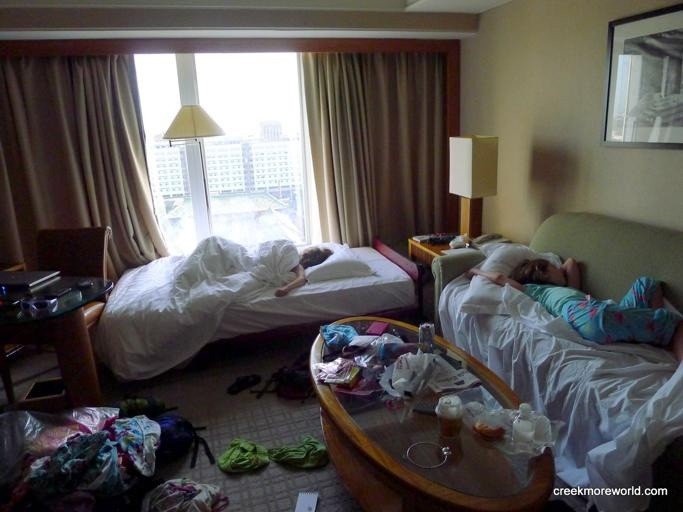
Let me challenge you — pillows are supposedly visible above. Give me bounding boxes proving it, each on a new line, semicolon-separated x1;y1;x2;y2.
300;237;377;285
457;237;564;317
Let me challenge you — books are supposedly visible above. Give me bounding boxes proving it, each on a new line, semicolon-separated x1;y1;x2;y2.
429;367;482;394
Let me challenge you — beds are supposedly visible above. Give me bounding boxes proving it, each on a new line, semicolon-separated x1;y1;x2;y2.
107;235;420;385
404;233;492;292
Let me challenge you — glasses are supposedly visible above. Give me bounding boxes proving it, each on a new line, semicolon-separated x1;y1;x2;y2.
20;297;57;312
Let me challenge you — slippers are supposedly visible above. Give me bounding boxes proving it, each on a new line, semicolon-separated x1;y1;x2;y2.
228;373;260;394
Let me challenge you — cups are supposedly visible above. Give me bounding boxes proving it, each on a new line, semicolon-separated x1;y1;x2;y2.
434;395;464;436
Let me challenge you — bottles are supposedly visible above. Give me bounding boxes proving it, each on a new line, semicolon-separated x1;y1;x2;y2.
511;403;535;446
378;342;431;361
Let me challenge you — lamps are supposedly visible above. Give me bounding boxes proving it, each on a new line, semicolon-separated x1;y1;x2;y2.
163;104;225;231
446;129;500;241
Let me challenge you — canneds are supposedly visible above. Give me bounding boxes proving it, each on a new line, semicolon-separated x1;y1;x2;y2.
418;323;436;353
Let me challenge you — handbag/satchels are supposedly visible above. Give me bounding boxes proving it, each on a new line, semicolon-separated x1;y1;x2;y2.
273;360;312;397
114;398;164;417
156;415;194;469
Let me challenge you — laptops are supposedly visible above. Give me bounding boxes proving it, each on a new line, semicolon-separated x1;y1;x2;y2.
0;270;61;288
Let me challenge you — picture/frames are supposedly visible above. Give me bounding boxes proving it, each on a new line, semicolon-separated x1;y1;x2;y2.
596;3;683;152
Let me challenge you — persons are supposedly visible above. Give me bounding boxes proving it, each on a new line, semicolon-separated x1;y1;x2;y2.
466;251;683;374
269;244;335;298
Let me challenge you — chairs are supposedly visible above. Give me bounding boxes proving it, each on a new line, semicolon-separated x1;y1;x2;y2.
1;224;114;352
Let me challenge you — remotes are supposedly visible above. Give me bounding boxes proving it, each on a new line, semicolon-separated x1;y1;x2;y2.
48;287;72;297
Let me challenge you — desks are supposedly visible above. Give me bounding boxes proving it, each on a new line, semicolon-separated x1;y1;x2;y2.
1;265;114;413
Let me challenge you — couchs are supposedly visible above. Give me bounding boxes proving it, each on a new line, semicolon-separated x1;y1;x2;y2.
432;208;683;505
